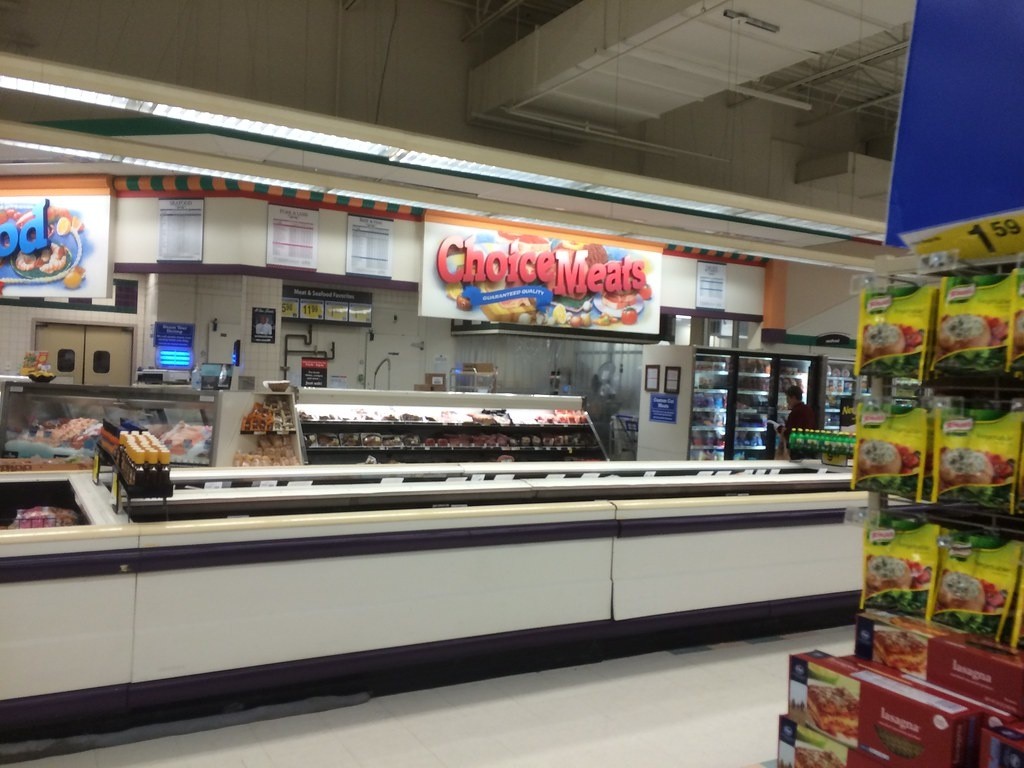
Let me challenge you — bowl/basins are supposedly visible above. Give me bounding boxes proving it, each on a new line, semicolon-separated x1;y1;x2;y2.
268;383;290;392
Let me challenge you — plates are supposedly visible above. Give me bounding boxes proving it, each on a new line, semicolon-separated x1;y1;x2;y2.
28;373;57;383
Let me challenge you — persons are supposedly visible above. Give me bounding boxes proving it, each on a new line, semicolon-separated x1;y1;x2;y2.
769;386;816;463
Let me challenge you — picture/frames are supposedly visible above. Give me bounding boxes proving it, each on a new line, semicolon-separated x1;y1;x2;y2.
664;366;681;394
645;365;660;392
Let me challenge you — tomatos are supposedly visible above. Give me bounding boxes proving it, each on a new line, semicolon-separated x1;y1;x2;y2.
640;283;652;299
622;307;637;324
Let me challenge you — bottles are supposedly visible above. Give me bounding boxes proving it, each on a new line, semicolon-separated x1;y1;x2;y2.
191;363;202;388
789;428;857;456
116;430;170;487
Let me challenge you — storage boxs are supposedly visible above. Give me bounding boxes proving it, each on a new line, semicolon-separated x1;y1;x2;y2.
777;613;1024;768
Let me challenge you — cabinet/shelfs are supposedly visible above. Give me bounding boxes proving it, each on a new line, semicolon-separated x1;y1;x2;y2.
240;386;610;491
